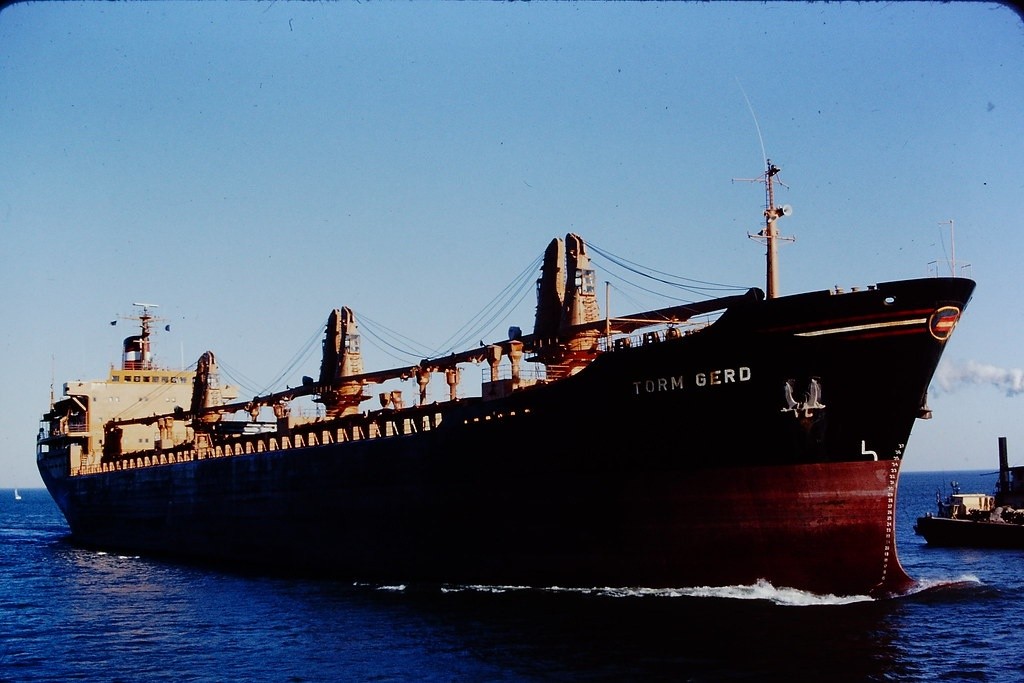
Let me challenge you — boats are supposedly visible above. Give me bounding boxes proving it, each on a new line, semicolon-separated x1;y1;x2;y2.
915;436;1024;550
36;154;977;604
